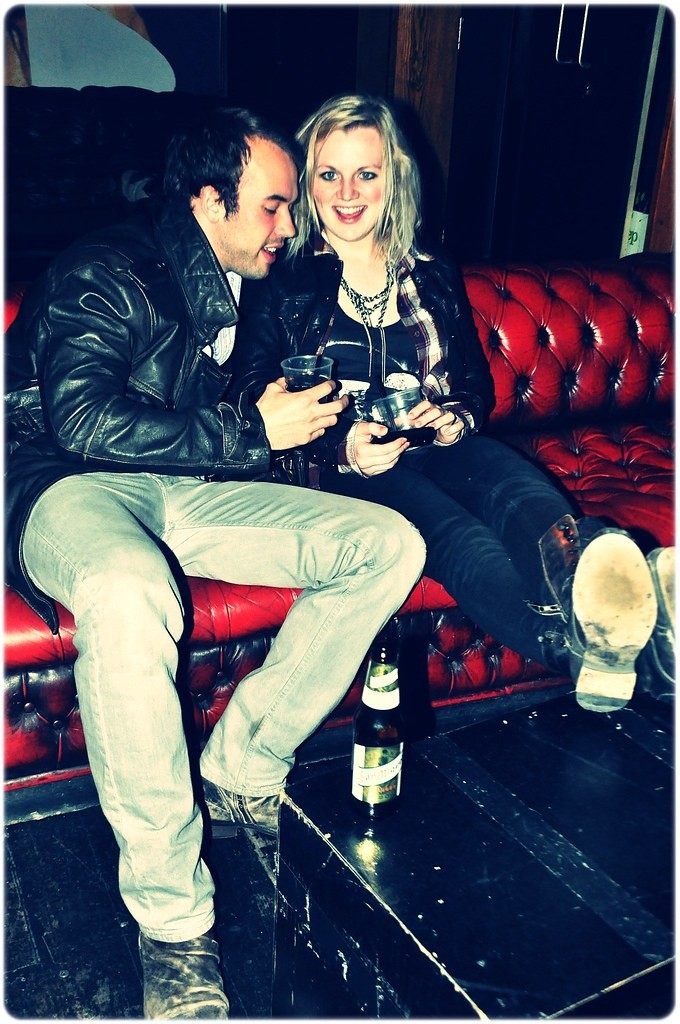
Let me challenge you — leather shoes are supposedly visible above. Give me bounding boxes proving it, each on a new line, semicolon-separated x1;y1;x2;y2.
138;932;230;1020
196;778;285;836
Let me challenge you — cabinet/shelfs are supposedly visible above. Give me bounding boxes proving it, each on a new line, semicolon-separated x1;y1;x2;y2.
442;7;674;267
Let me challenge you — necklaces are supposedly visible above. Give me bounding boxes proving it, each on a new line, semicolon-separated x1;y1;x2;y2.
340;260;394;329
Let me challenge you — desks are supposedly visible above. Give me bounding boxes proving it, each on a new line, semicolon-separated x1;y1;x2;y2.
271;690;675;1019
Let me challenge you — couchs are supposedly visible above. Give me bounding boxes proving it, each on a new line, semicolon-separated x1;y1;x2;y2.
3;250;675;782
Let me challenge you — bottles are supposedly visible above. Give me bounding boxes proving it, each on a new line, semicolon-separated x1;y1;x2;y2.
350;643;403;821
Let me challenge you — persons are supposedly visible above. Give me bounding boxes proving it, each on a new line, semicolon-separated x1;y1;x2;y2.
237;93;675;712
4;106;426;1019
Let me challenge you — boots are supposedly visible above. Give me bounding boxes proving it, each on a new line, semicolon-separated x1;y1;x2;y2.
647;543;676;703
541;514;657;715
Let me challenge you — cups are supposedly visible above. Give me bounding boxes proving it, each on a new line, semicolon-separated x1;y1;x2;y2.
280;354;334;404
372;387;424;452
